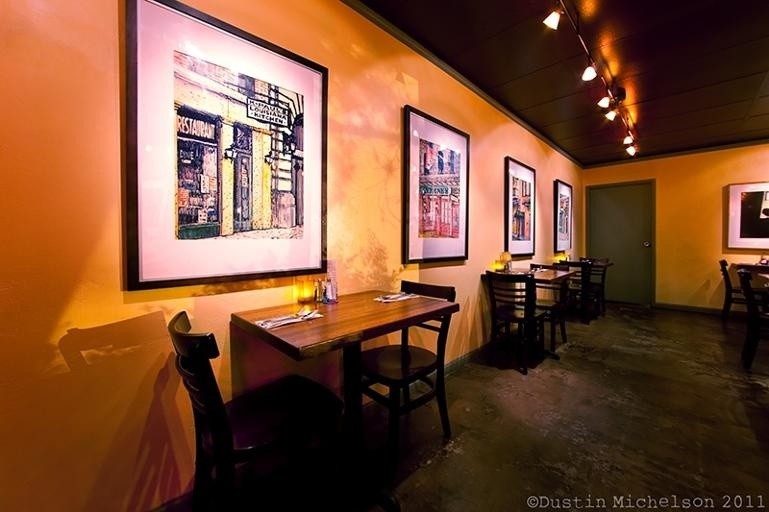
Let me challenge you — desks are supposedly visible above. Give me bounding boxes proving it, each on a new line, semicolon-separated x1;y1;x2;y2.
731;262;769;272
757;273;769;280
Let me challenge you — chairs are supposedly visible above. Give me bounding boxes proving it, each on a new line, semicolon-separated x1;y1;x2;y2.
718;259;761;324
736;268;769;370
168;256;614;512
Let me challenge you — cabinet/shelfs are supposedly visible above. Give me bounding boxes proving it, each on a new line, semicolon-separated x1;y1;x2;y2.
553;179;574;254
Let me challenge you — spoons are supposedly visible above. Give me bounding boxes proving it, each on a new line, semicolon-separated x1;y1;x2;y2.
260;307;319;330
379;292;416;301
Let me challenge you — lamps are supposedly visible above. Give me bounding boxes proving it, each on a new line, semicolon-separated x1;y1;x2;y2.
542;0;637;157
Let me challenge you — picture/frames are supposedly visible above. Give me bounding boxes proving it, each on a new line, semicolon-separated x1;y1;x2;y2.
503;156;537;258
726;181;769;250
401;104;471;265
124;0;330;292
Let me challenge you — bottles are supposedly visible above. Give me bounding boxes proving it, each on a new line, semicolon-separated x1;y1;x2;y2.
314;277;333;302
